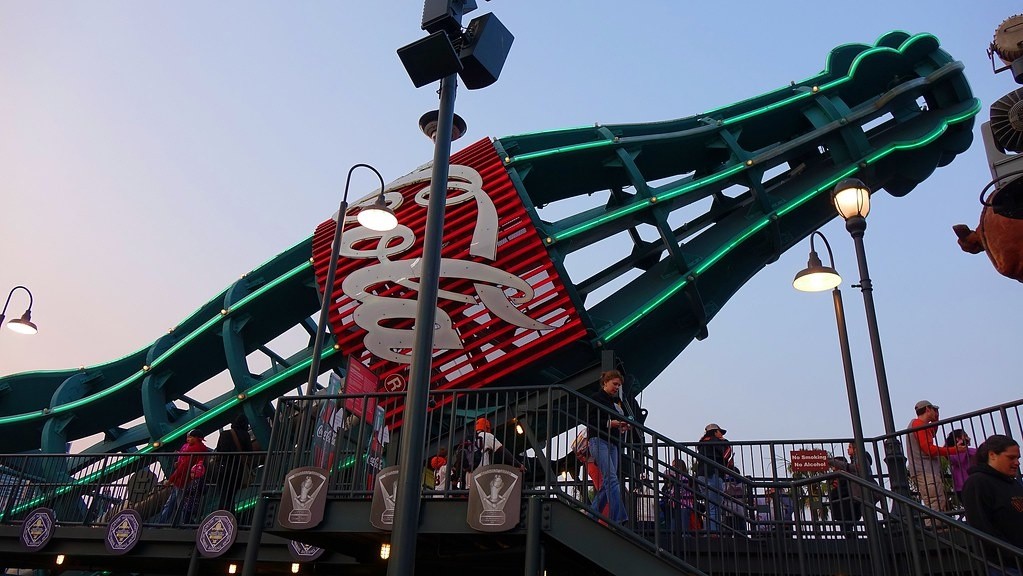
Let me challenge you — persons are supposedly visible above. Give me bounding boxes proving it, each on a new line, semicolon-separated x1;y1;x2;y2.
906;401;967;532
830;443;879;538
944;429;978;501
961;434;1023;576
158;415;253;524
578;370;628;524
434;418;528;498
661;424;794;538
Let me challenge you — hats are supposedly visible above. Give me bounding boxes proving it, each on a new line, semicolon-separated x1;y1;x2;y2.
190;464;206;479
188;429;206;441
476;418;491;432
431;457;447;468
915;400;939;411
706;424;727;435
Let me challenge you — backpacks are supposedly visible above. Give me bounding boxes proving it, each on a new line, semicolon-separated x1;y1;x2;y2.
456;430;489;473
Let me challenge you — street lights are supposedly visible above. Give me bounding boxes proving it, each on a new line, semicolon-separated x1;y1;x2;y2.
389;0;516;576
0;285;39;335
791;230;885;576
830;178;924;576
239;163;400;576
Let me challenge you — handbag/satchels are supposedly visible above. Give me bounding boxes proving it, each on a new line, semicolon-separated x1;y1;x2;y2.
243;467;256;485
571;428;592;462
